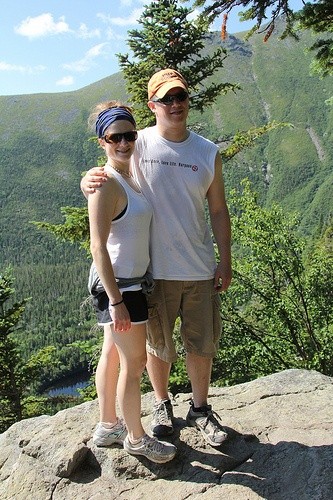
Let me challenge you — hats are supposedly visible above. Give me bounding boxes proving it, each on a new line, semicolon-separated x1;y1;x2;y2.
148;68;188;101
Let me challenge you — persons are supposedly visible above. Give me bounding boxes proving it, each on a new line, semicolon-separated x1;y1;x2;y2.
88;101;177;463
80;68;232;446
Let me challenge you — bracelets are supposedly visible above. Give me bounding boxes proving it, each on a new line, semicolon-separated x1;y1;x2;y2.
110;300;123;306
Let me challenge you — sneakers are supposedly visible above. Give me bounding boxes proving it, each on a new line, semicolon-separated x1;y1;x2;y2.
186;400;229;447
151;399;174;435
123;433;176;464
93;418;129;446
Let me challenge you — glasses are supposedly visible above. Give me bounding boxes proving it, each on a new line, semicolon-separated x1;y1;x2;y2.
154;92;189;105
101;131;138;143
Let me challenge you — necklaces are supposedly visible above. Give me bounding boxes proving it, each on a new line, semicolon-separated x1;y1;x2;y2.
105;163;131;177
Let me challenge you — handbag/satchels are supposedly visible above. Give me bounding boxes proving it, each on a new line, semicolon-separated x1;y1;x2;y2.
89;271;155;296
